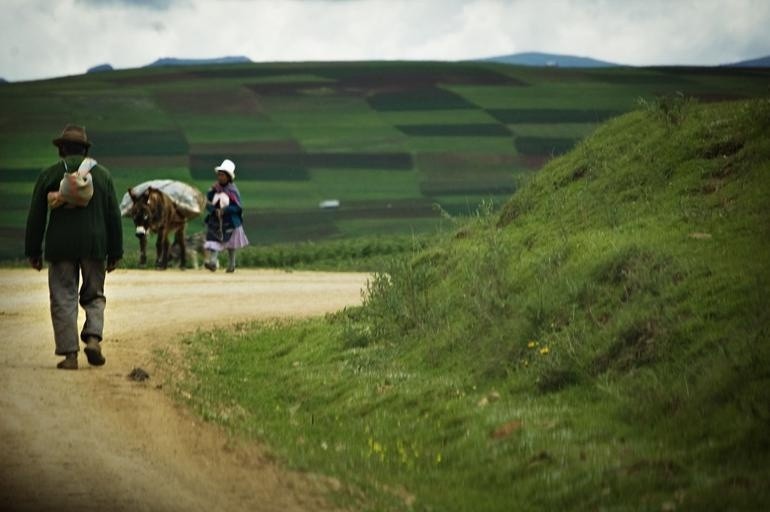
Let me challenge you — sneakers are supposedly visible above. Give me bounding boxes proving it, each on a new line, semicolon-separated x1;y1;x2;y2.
57;357;77;369
84;342;105;365
204;262;235;272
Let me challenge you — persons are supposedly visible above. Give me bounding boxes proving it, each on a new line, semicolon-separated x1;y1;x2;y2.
203;158;249;272
25;127;124;370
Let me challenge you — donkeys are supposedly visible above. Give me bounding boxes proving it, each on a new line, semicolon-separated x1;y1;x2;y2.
126;186;187;270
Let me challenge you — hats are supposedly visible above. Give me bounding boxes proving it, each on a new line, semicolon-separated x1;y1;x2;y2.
53;122;91;146
215;159;236;180
